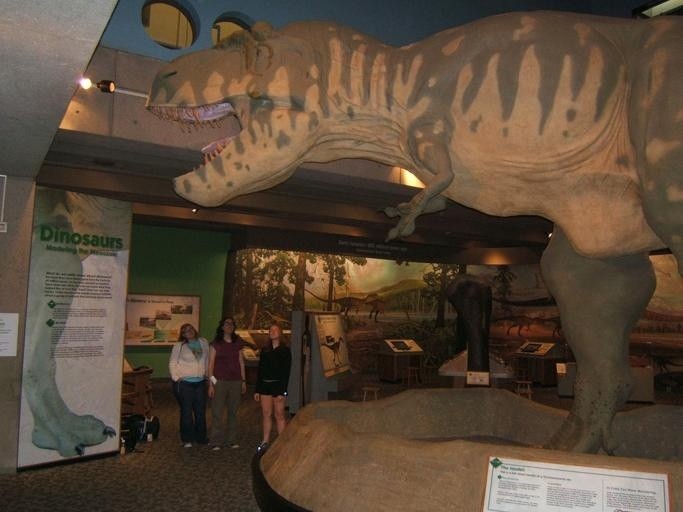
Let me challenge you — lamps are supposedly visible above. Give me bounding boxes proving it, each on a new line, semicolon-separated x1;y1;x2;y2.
96;80;116;93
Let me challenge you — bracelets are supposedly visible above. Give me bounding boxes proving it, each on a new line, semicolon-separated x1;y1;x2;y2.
242;380;247;382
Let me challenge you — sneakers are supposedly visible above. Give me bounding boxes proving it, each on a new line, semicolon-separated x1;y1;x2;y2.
182;437;275;453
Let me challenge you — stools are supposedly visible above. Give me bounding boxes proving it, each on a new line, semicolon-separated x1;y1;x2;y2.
359;386;383;401
405;367;421;386
513;380;535;400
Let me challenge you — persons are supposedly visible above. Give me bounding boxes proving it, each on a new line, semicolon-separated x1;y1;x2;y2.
170;323;210;448
252;323;291;448
207;316;246;452
